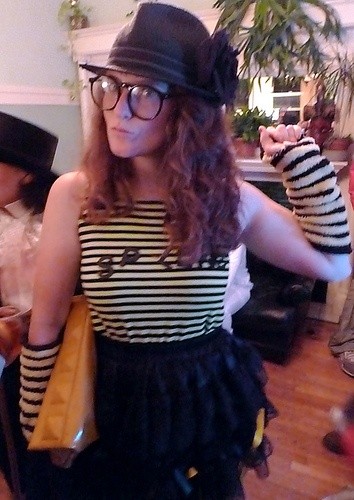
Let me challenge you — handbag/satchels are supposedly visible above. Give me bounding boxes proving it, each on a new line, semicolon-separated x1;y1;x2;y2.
0;294;98;453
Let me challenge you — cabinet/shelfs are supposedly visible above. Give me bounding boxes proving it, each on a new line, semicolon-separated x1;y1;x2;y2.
271;76;315;121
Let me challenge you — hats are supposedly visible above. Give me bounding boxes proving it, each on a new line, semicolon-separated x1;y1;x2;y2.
0;111;58;172
79;3;239;105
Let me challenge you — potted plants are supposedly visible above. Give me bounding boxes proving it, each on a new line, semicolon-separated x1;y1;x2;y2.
227;107;276;158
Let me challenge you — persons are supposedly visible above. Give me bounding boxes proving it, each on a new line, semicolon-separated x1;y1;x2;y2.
17;3;354;500
0;112;66;500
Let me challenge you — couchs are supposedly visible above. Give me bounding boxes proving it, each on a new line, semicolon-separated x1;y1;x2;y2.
232;181;318;365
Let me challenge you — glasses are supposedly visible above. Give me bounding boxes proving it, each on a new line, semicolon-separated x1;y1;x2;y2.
89;74;187;121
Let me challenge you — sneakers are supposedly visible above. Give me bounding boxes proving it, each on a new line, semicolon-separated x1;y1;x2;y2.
337;351;354;377
